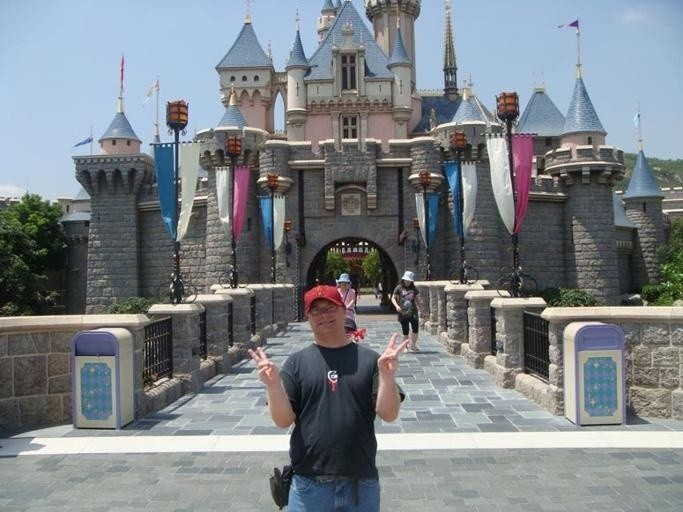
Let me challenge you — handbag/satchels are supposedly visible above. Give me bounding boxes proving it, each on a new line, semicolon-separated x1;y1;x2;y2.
269;466;294;510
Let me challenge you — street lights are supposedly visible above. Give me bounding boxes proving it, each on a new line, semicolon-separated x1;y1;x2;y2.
165;101;188;304
266;172;278;281
420;171;430;282
495;91;520;297
224;135;241;290
449;131;467;285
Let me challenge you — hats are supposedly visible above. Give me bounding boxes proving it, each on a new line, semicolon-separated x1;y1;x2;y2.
335;273;351;286
401;271;415;281
302;285;344;315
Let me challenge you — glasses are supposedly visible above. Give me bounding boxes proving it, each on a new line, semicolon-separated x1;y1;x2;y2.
308;303;337;313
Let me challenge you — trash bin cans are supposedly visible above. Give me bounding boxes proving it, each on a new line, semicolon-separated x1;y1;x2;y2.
563;320;626;427
70;325;135;430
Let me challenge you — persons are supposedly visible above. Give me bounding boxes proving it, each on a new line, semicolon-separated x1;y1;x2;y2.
246;284;408;512
335;273;365;342
390;270;424;352
